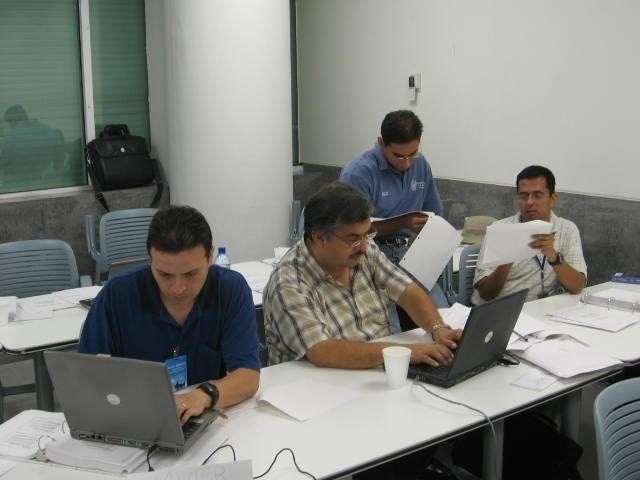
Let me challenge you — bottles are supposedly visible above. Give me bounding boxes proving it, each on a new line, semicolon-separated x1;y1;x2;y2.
214;247;232;269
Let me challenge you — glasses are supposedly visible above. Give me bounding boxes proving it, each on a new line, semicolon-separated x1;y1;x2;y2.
396;155;420;159
323;224;377;246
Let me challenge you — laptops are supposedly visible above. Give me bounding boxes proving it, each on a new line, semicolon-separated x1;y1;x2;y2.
383;288;529;388
42;350;221;456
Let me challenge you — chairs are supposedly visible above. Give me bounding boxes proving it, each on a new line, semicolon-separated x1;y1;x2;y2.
2;208;158;300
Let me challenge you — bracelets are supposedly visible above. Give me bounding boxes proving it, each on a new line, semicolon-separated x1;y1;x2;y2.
430;323;451;341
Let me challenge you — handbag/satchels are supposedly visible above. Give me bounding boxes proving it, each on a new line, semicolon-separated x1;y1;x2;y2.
85;124;155;190
452;413;582;479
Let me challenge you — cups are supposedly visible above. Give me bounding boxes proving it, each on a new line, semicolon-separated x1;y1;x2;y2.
380;345;412;390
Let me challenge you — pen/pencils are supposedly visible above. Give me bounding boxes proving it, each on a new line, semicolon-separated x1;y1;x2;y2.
219;412;228;420
513;330;528;343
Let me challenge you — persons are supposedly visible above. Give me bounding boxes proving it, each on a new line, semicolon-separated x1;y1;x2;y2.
466;164;588;307
261;180;464;479
4;104;64;150
79;204;260;426
338;110;462;336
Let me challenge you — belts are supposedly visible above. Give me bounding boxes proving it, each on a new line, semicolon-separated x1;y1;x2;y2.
373;239;415;245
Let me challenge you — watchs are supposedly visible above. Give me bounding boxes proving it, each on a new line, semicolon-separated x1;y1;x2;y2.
196;382;219;409
547;251;565;265
